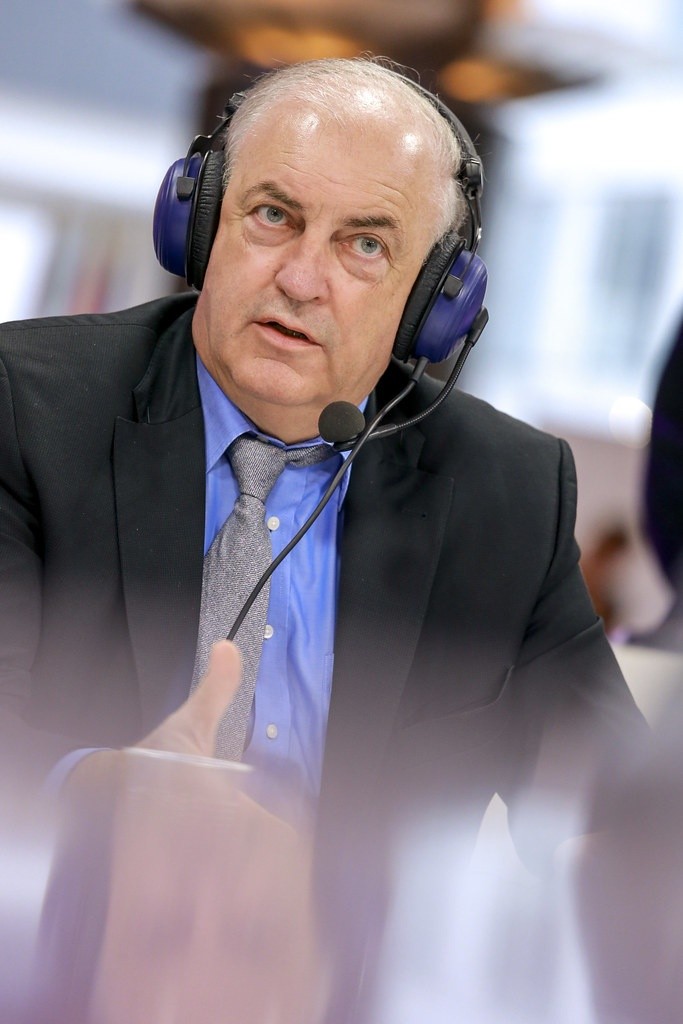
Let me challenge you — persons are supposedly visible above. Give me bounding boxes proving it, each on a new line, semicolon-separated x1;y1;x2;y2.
3;58;657;866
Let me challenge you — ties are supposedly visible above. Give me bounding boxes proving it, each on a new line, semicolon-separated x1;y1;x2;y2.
189;437;338;762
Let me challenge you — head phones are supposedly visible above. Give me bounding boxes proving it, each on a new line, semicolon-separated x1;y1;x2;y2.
152;64;489;452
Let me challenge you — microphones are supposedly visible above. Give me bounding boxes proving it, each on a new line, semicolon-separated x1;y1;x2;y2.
317;400;399;452
